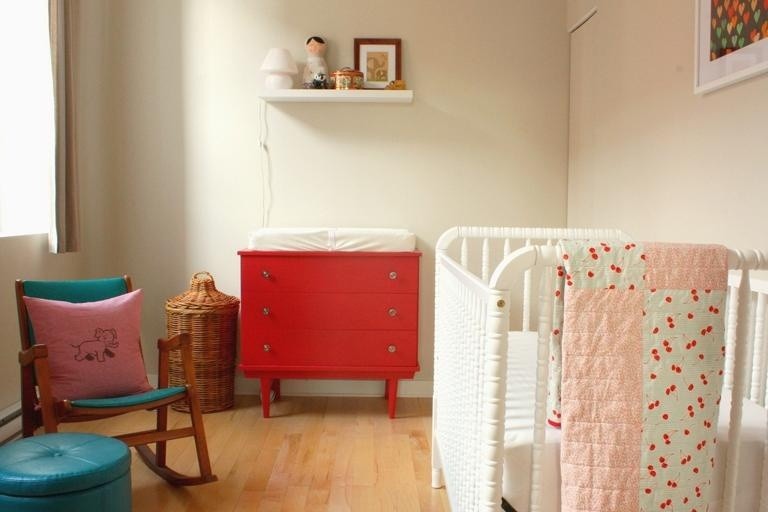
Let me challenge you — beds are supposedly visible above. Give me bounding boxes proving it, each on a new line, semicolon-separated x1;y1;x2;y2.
433;226;768;512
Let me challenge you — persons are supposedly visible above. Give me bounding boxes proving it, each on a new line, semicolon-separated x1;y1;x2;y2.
300;32;329;88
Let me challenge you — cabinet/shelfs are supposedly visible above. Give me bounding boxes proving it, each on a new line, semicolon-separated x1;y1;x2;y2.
238;250;421;419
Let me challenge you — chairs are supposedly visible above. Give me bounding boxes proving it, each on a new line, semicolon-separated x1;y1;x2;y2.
15;277;216;485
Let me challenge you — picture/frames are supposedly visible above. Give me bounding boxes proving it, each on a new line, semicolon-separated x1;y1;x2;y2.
354;37;402;87
692;0;768;95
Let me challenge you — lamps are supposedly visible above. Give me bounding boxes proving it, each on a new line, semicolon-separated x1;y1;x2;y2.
260;46;296;88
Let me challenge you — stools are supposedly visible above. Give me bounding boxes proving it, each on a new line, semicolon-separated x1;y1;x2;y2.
0;433;130;512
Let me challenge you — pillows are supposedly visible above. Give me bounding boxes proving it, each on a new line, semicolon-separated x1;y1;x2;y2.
21;287;155;395
